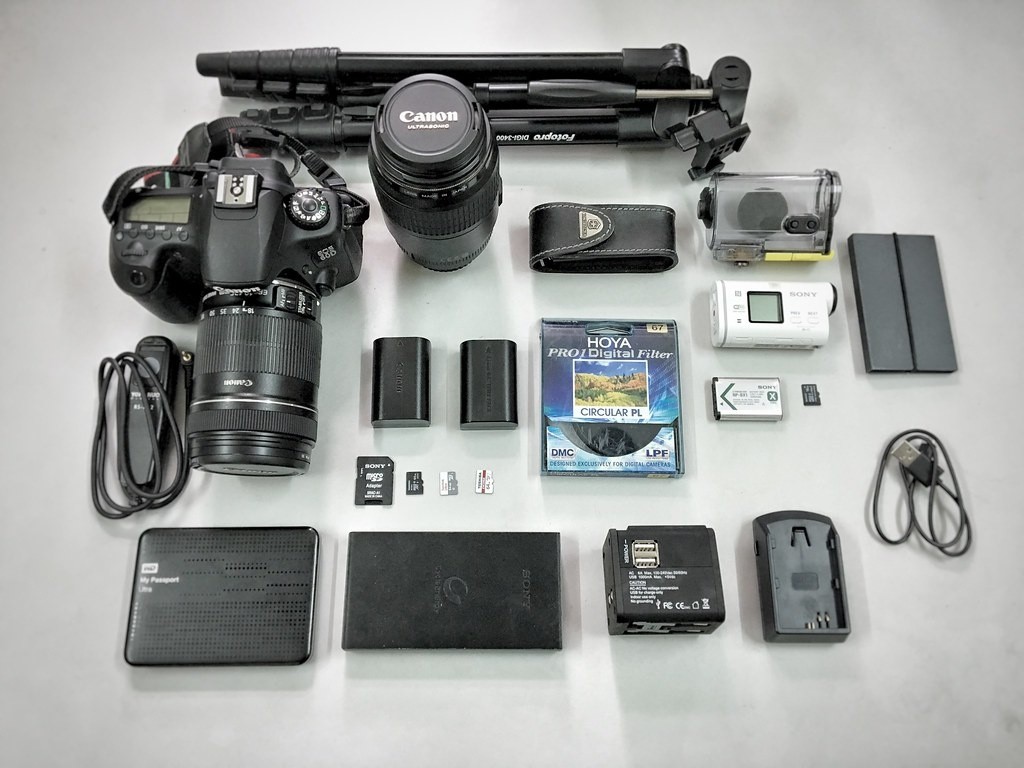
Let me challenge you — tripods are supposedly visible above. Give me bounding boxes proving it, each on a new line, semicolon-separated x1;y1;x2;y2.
183;47;752;182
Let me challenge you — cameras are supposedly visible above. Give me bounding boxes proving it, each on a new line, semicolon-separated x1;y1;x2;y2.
109;159;365;477
